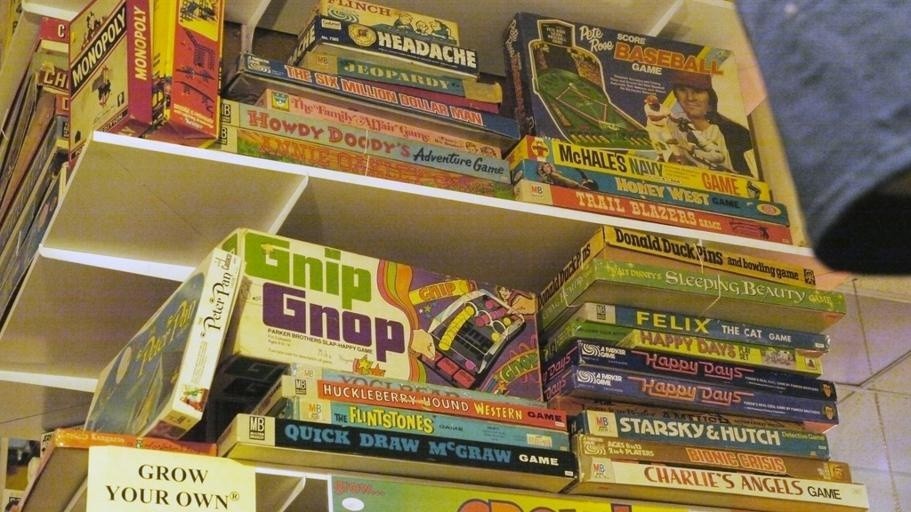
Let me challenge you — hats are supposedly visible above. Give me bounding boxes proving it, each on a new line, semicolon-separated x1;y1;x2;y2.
671;65;711;91
643;94;659;106
